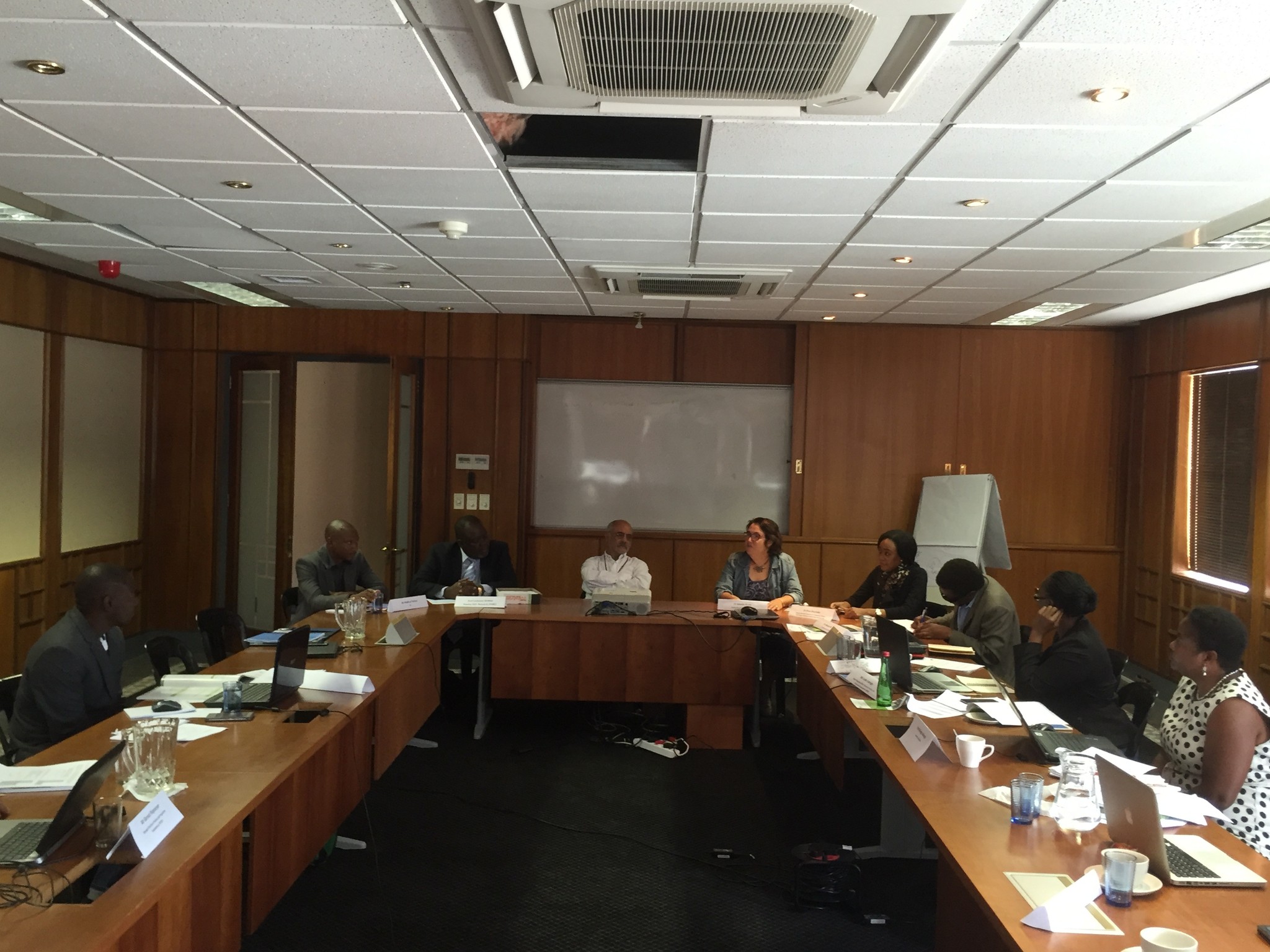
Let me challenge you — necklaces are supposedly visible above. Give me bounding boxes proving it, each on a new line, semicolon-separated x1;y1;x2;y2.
1196;670;1238;700
604;555;628;573
751;559;769;573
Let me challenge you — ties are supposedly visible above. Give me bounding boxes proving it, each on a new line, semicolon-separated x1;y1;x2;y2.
464;560;477;581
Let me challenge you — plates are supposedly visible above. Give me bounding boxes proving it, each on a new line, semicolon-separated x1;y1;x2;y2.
1120;946;1142;952
965;712;999;724
1084;864;1163;896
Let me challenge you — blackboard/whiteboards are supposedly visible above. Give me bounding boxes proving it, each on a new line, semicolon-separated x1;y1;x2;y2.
532;377;794;537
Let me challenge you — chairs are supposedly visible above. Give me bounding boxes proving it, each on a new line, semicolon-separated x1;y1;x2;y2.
195;607;248;666
1116;681;1159;760
144;635;200;687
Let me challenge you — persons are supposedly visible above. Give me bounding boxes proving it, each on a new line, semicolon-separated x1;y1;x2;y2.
580;519;653;600
288;520;387;626
1148;602;1270;858
714;517;803;715
911;558;1021;688
410;516;517;695
1013;570;1135;753
830;529;927;620
8;563;142;762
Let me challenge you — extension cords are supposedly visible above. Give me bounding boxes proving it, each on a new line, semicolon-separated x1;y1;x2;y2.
633;737;680;759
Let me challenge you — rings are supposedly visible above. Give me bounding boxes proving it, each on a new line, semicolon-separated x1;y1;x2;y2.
459;590;462;592
472;593;475;596
473;587;476;590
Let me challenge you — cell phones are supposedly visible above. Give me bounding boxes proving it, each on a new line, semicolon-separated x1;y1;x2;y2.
960;699;998;704
205;712;254;721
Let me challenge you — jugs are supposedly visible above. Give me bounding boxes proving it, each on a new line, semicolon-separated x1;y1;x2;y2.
860;614;880;659
1053;746;1101;832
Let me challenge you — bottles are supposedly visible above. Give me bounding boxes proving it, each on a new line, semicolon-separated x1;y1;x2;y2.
876;650;892;706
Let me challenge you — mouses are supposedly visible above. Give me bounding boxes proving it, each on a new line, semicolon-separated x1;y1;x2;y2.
741;606;757;616
152;699;181;712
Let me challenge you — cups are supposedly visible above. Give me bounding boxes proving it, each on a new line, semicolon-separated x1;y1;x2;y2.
372;593;383;613
120;717;179;794
841;638;862;661
92;796;122;852
836;635;850;659
223;681;243;713
334;598;366;639
1010;779;1037;825
955;734;994;768
1104;851;1137;907
1018;772;1044;818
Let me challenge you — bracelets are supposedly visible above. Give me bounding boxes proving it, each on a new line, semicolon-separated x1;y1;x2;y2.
477;588;482;596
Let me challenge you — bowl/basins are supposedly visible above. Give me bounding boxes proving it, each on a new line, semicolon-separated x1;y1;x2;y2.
1140;927;1198;952
1100;848;1149;886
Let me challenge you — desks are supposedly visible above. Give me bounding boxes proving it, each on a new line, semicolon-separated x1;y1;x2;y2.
0;598;1270;952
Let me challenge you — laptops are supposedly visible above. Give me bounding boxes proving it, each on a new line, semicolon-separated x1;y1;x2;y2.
983;667;1128;765
1096;754;1266;886
203;625;311;708
0;737;127;868
876;614;973;694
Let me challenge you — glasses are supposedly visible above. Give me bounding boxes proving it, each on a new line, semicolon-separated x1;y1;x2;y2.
1033;594;1047;601
941;593;963;604
743;531;765;541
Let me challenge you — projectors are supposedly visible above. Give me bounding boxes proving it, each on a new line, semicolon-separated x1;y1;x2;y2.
591;588;651;615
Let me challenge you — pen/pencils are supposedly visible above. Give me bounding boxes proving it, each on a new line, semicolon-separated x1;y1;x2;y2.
920;607;927;623
956;693;982;696
